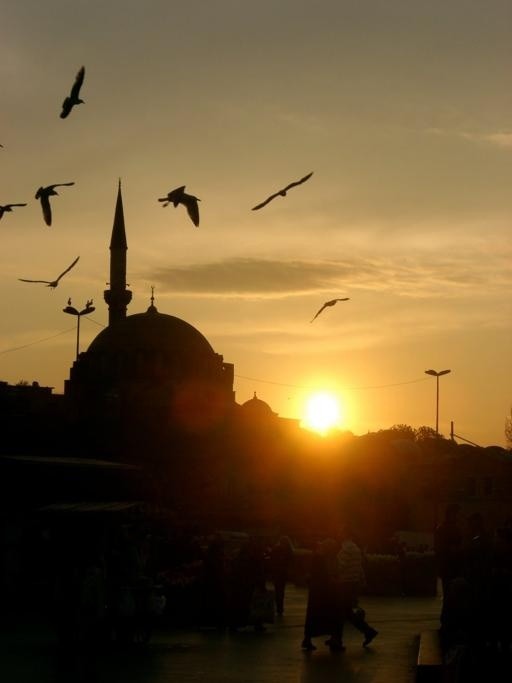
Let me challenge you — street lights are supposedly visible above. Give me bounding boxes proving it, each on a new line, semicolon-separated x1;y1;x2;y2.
427;369;451;433
64;307;96;358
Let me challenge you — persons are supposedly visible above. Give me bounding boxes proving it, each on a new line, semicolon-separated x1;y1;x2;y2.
206;529;379;654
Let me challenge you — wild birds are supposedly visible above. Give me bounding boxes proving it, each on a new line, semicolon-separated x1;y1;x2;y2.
86;299;93;309
59;65;86;119
251;172;312;210
35;182;75;226
0;204;27;220
68;297;71;305
310;298;349;322
158;185;201;227
18;255;80;287
